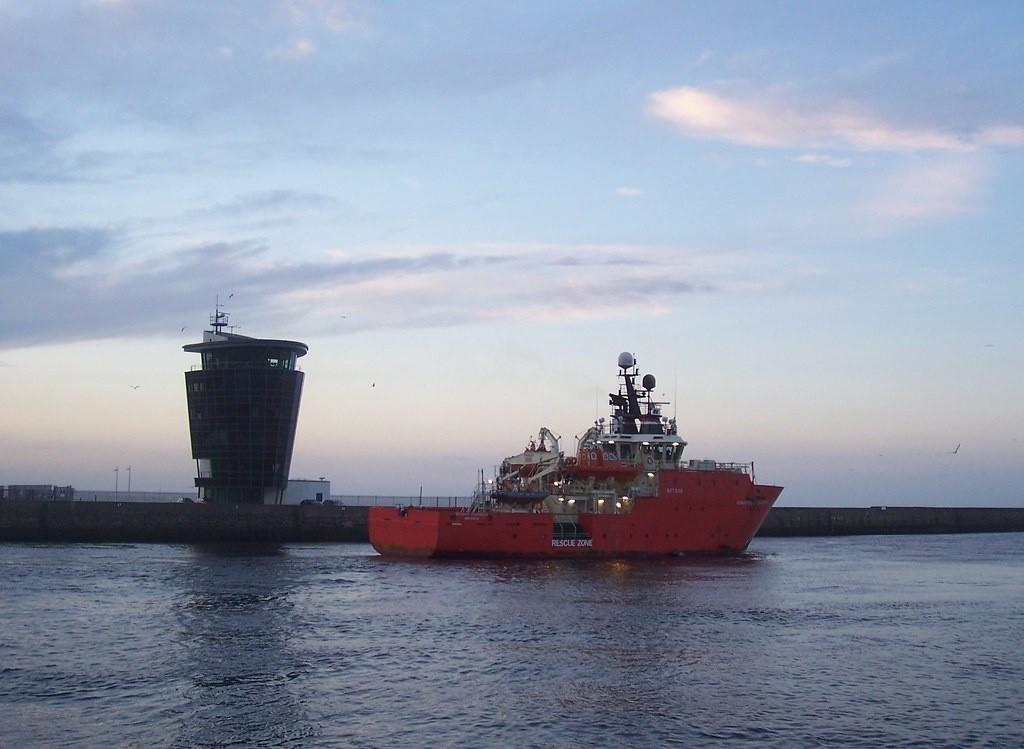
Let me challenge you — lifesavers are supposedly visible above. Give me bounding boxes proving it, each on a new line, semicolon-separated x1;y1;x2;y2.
648;457;653;465
565;457;577;464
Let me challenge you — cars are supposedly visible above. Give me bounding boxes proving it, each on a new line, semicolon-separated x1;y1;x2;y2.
176;497;196;504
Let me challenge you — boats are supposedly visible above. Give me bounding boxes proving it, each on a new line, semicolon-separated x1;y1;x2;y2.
368;348;784;560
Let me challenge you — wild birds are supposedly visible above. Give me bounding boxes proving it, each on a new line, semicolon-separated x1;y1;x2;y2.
950;444;960;454
131;385;141;389
